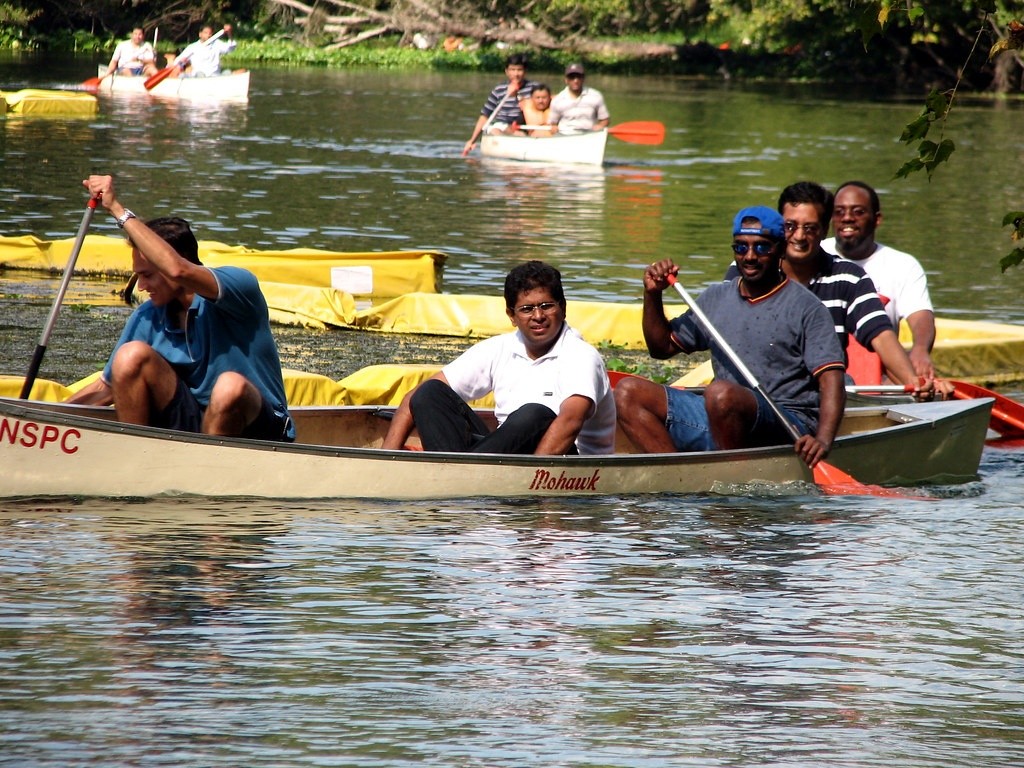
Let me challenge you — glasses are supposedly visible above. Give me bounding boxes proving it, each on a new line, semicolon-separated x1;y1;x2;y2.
731;244;780;256
783;221;825;234
831;206;875;222
509;303;562;318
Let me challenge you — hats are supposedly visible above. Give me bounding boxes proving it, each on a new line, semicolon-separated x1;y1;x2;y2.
732;205;785;237
565;63;585;76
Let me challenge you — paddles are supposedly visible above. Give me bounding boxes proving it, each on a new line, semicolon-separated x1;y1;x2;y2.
144;28;227;91
667;273;858;486
20;191;104;401
511;120;666;145
670;376;928;394
82;47;149;86
937;377;1024;438
462;85;517;156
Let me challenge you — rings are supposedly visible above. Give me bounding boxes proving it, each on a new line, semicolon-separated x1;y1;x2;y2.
930;390;933;398
652;262;656;267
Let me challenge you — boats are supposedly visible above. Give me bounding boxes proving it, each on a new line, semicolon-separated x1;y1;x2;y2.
0;390;996;507
480;125;610;168
96;63;251;106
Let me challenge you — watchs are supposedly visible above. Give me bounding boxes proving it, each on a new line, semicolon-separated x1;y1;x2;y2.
117;208;136;229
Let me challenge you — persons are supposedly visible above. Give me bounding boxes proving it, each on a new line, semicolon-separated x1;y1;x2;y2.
507;84;558;138
381;259;618;457
172;23;247;79
546;63;611;137
723;182;937;402
613;205;846;470
820;181;956;399
60;173;296;442
460;54;545;157
107;27;159;77
